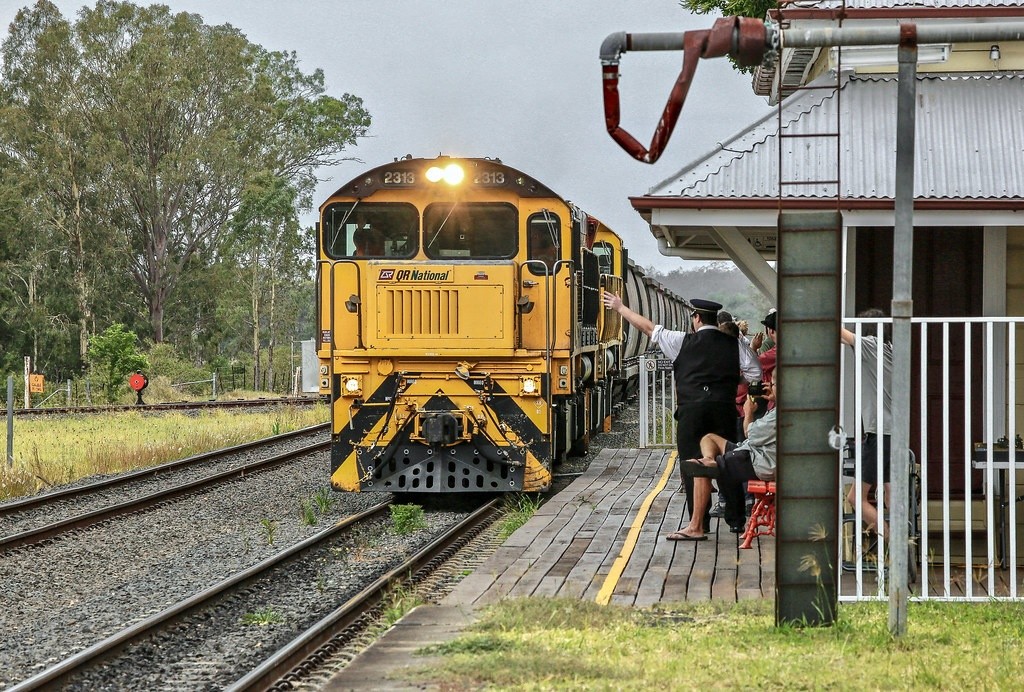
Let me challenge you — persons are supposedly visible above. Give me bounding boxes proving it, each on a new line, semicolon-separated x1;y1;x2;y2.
351;227;386;256
842;309;892;582
600;290;777;541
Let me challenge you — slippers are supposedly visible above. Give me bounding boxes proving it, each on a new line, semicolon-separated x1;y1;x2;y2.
679;459;721;480
666;532;709;541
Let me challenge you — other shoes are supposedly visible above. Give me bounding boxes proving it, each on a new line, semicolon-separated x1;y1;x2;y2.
709;503;726;517
730;527;745;533
745;504;753;516
874;568;890;583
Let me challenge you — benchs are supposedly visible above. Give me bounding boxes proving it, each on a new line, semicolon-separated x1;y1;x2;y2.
739;482;776;550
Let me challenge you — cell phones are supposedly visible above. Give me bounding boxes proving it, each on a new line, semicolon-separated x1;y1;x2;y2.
749;386;766;396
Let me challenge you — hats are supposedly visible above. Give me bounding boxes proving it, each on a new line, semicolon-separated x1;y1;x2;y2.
761;312;776;330
690;299;723;317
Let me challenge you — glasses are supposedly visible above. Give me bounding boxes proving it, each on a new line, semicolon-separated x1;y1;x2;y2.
769;382;774;387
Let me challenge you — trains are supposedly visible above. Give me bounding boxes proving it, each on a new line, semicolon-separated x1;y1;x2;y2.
313;152;703;496
